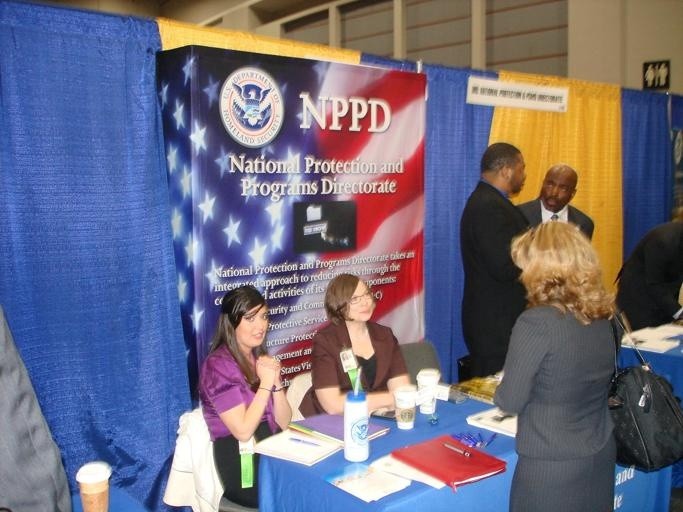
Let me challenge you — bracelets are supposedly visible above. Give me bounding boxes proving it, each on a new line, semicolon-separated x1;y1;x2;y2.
257;386;273;393
273;384;286;393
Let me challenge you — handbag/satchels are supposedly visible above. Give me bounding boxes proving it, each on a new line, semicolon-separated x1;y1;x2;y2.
608;315;683;474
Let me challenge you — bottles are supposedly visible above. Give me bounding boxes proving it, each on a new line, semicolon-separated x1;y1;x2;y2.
342;390;369;462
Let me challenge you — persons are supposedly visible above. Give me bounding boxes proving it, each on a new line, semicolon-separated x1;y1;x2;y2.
460;142;531;377
494;219;625;512
612;210;683;332
0;301;72;512
298;273;411;414
197;285;293;509
514;163;594;244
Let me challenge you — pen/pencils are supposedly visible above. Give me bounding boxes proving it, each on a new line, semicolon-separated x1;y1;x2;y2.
440;441;471;458
290;438;321;447
453;433;498;449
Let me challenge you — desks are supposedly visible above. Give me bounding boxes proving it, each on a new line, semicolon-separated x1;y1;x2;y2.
617;319;683;490
256;368;673;512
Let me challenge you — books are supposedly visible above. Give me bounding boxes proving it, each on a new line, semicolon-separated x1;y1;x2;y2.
451;375;510;405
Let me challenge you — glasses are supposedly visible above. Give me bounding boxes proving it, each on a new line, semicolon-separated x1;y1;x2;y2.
349;292;374;303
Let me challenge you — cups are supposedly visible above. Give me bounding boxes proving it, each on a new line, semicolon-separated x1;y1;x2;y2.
392;384;416;430
416;368;440;414
76;461;112;512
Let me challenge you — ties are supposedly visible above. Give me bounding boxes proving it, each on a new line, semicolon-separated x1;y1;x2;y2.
551;214;558;221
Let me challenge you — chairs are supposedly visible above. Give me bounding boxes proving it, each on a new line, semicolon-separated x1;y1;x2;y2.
178;407;259;512
399;341;445;384
285;369;327;425
